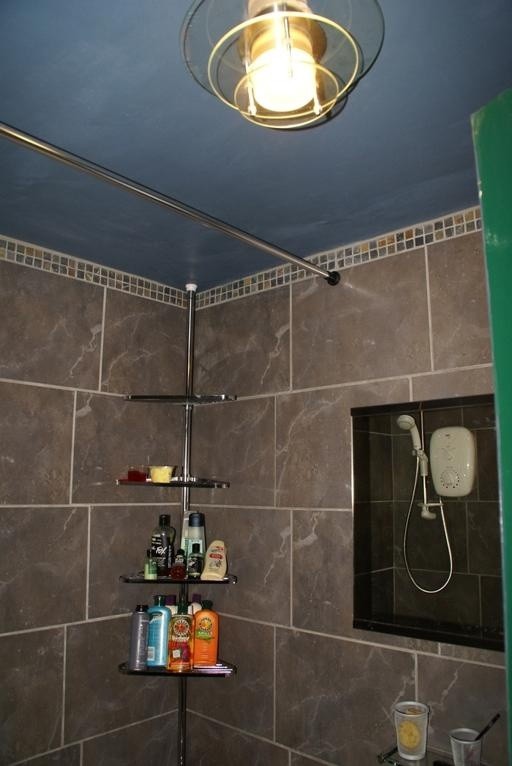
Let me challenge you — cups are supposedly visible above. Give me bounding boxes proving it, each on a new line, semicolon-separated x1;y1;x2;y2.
448;728;483;766
127;464;175;483
391;701;429;762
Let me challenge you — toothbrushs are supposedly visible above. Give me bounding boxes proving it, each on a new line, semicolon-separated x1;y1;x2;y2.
464;712;502;762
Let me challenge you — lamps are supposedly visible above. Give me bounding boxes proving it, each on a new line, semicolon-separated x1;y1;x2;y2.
180;0;386;131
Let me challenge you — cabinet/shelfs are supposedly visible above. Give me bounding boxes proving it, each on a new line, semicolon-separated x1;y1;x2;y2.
115;284;238;765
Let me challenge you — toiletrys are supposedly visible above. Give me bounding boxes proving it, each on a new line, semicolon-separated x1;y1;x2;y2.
128;511;227;672
